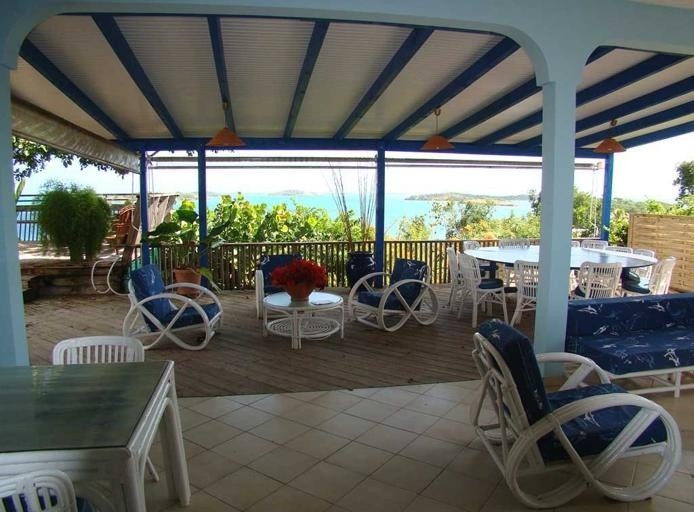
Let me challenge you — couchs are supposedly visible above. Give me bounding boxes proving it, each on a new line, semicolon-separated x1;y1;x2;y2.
565;292;694;398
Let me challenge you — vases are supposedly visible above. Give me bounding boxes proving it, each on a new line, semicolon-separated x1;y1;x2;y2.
284;286;314;301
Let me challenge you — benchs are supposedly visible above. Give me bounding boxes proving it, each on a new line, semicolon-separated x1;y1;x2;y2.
106;204;134;256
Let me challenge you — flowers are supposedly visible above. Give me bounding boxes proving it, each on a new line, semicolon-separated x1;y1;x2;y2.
270;259;328;291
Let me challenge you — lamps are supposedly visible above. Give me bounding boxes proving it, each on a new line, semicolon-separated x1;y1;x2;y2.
420;108;456;150
206;98;247;147
593;118;626;153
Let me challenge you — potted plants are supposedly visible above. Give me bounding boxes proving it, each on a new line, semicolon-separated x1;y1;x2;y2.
140;207;231;298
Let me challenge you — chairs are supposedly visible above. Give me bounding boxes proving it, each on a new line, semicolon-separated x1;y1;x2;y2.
606;247;634;253
255;251;302;319
499;238;535;284
52;335;159;484
471;319;683;509
348;258;439;332
622;256;676;295
628;249;654;282
457;254;509;328
122;264;224;351
1;469;93;512
571;240;580;248
570;261;622;299
581;239;608;250
463;241;481;248
510;260;539;328
446;247;486;313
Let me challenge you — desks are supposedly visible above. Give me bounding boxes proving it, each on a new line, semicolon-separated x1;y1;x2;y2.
0;360;191;512
463;245;658;300
263;291;345;350
